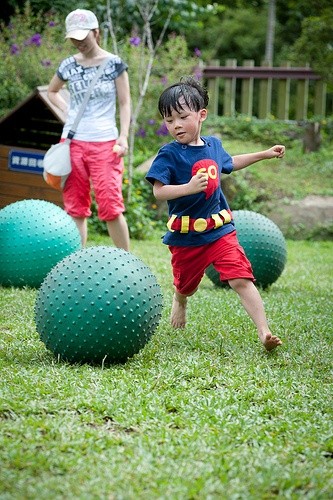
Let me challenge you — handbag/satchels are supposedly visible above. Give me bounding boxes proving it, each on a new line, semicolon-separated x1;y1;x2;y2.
43;142;72;191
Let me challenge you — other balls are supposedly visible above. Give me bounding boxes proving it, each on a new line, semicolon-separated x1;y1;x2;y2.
205;210;287;289
0;199;83;289
34;245;164;366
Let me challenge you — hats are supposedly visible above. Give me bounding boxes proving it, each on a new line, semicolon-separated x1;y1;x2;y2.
64;9;99;41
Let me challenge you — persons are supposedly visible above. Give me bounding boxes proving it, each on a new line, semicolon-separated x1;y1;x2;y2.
142;77;288;351
46;8;135;252
